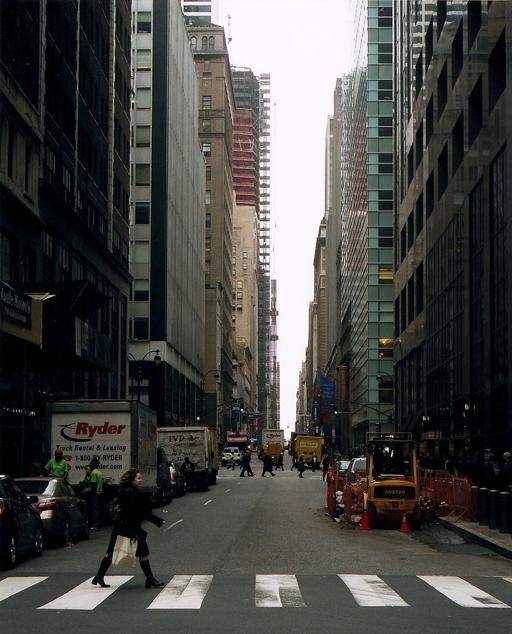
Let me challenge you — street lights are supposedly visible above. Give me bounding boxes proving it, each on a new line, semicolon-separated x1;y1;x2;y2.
128;349;160;404
195;367;226;423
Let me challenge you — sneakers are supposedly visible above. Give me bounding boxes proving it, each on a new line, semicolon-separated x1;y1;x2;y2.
298;475;304;478
240;474;254;477
261;474;275;476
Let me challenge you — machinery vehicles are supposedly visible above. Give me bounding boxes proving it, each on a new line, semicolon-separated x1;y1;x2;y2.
364;430;420;528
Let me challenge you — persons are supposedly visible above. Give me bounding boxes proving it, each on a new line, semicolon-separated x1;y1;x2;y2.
80;465;94;530
92;470;165;589
226;448;330;481
181;457;196;492
90;459;103;530
43;450;68;480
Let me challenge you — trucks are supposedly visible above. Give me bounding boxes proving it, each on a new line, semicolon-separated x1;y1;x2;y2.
260;429;284;464
155;425;220;491
294;435;322;465
50;399;171;521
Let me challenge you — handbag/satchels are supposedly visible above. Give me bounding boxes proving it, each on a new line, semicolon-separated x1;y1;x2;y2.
112;535;137;569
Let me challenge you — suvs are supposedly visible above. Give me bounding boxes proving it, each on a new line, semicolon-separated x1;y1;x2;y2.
221;446;241;466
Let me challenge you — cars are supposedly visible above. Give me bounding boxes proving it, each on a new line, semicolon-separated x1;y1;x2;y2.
335;460;350;471
0;475;45;568
13;477;90;545
346;457;369;473
167;460;187;495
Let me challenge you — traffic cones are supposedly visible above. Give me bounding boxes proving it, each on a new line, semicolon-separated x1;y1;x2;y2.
399;513;409;533
361;510;371;530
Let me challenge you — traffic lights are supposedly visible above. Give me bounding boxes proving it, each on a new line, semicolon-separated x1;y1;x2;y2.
333;409;339;419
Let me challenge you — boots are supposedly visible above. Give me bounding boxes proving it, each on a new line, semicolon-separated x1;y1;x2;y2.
91;557;111;587
139;560;164;589
